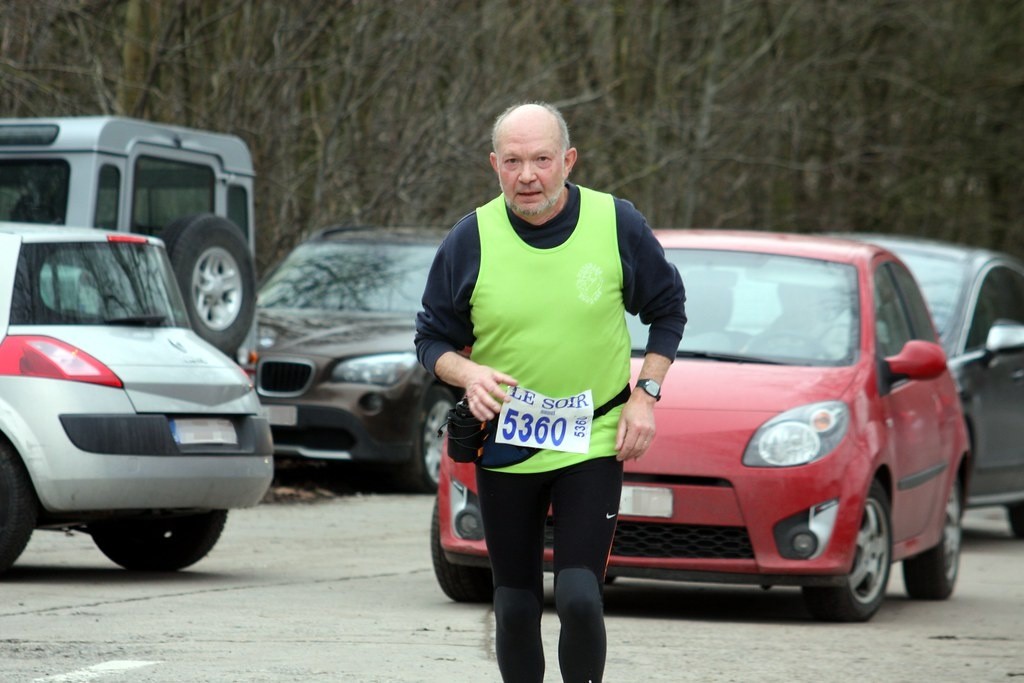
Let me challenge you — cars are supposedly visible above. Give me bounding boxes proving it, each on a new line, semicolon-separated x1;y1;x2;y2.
726;229;1024;542
423;224;973;624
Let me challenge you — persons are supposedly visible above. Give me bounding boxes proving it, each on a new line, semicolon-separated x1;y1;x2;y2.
414;100;687;683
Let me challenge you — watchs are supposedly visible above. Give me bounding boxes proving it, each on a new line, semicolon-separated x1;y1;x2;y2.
635;378;661;401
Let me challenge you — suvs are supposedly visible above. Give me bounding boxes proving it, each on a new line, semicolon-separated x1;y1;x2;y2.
0;114;261;395
0;220;275;577
234;223;454;495
